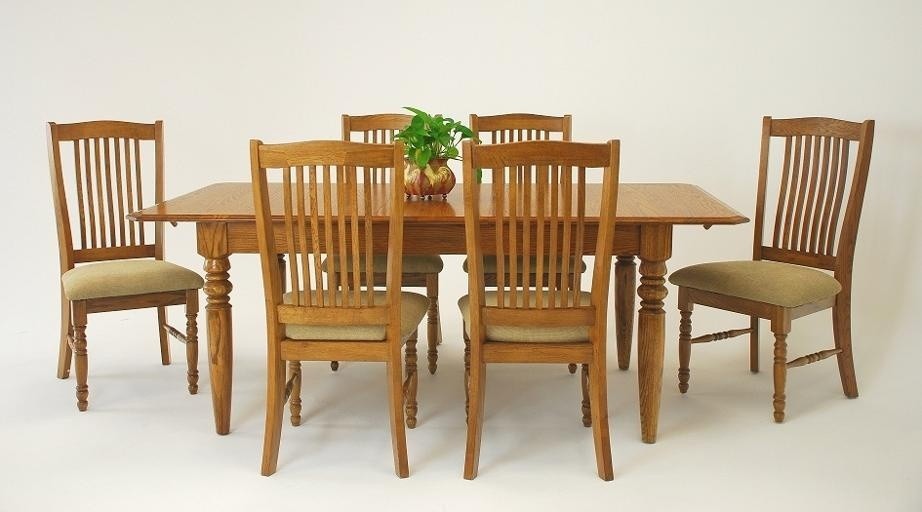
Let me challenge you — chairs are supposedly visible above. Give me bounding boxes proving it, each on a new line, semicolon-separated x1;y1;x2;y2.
462;138;622;479
47;120;204;411
249;139;431;478
669;117;876;424
321;115;445;374
462;112;586;374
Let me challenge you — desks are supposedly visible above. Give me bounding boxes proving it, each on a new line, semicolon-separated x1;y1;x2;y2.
126;182;751;444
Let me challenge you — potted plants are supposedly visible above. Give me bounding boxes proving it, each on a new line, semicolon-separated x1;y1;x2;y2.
391;107;483;200
